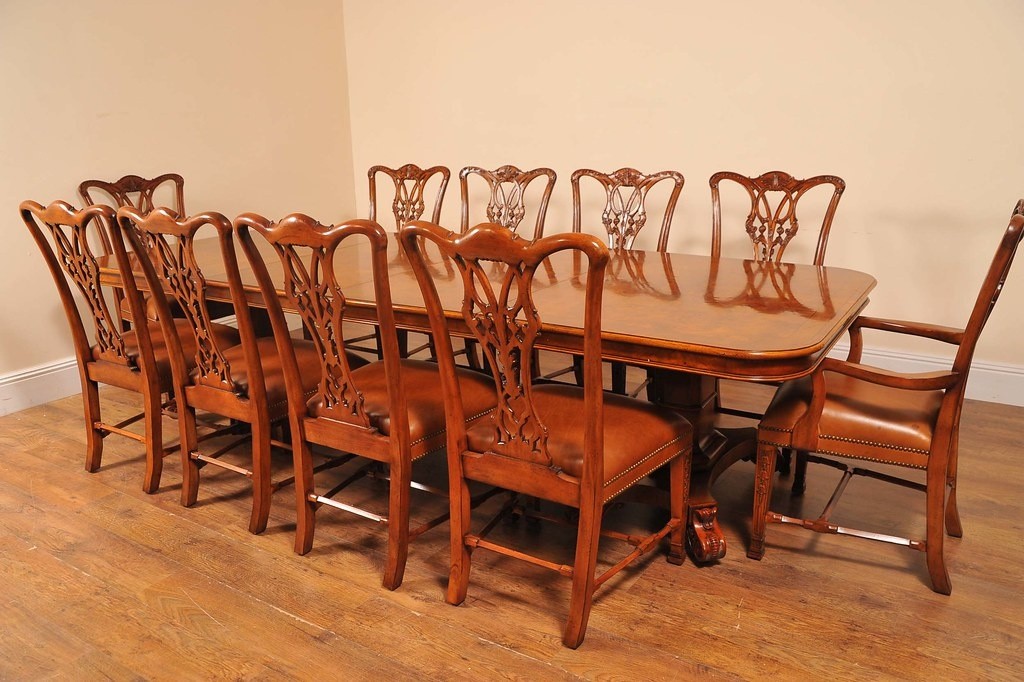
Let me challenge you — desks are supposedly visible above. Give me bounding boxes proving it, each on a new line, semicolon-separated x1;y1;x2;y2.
93;231;878;564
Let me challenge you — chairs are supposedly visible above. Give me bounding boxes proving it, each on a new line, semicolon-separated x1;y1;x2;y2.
747;198;1024;595
19;164;846;649
